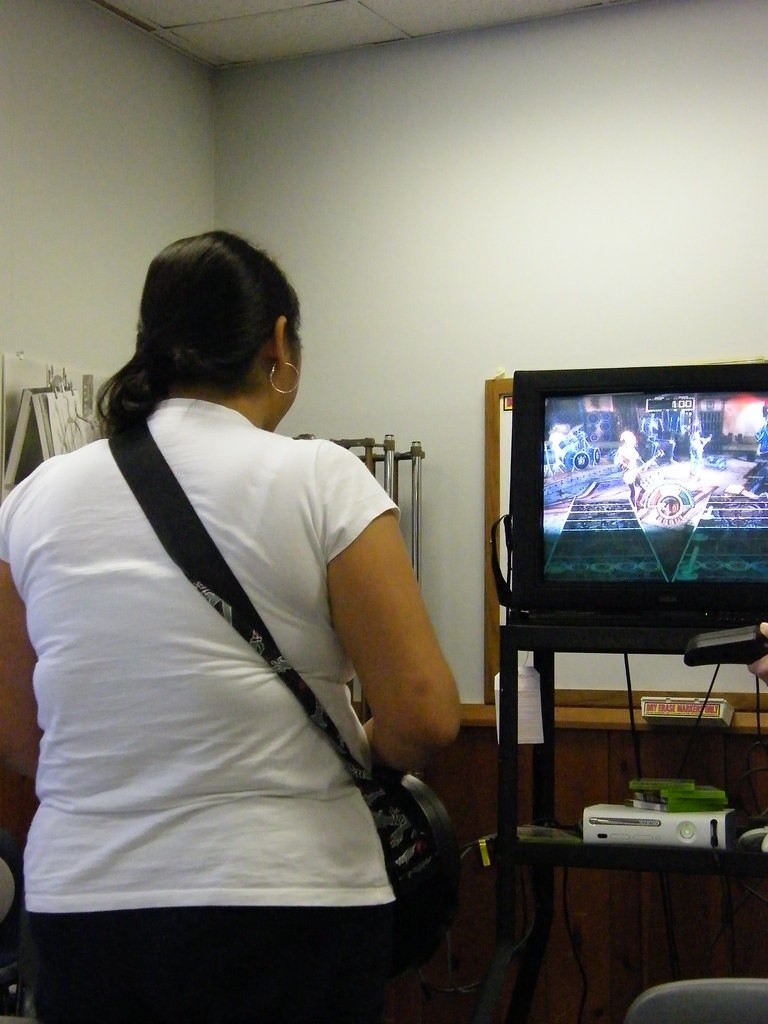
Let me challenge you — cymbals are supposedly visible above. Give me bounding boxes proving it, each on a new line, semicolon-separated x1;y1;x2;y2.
571;424;584;434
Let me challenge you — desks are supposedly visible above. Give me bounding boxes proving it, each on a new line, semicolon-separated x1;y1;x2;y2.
472;614;768;1024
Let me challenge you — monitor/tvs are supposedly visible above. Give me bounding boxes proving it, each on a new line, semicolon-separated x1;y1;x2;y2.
506;362;767;625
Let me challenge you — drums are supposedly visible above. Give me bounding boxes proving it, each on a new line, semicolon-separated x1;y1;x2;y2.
577;432;589;442
563;450;591;471
587;446;601;466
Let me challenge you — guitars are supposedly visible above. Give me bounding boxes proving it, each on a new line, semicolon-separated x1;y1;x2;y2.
368;770;459;972
692;434;713;458
621;449;666;485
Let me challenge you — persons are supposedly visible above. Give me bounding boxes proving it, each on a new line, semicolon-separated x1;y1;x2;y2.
0;230;462;1024
